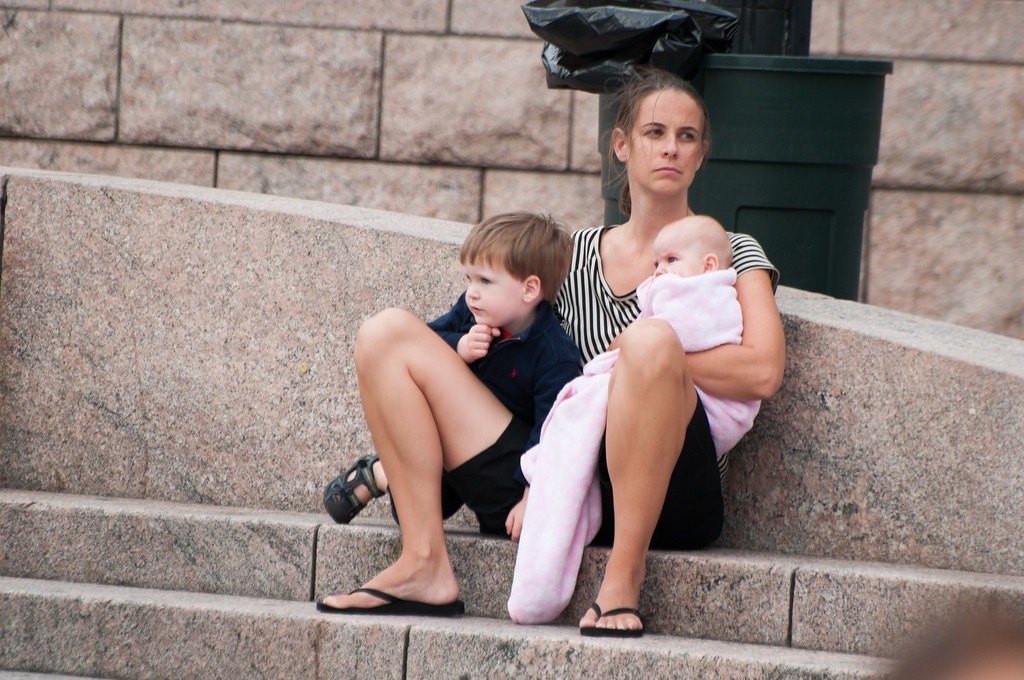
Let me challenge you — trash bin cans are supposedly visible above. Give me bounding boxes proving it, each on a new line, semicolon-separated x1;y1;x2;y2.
595;46;895;304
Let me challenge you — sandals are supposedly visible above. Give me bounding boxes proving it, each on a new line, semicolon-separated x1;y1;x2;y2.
323;454;387;526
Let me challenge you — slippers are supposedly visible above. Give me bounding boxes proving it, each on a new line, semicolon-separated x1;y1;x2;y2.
579;604;644;638
316;584;466;617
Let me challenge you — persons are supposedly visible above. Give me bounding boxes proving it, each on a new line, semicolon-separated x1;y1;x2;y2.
323;212;587;542
316;70;787;636
651;215;763;458
